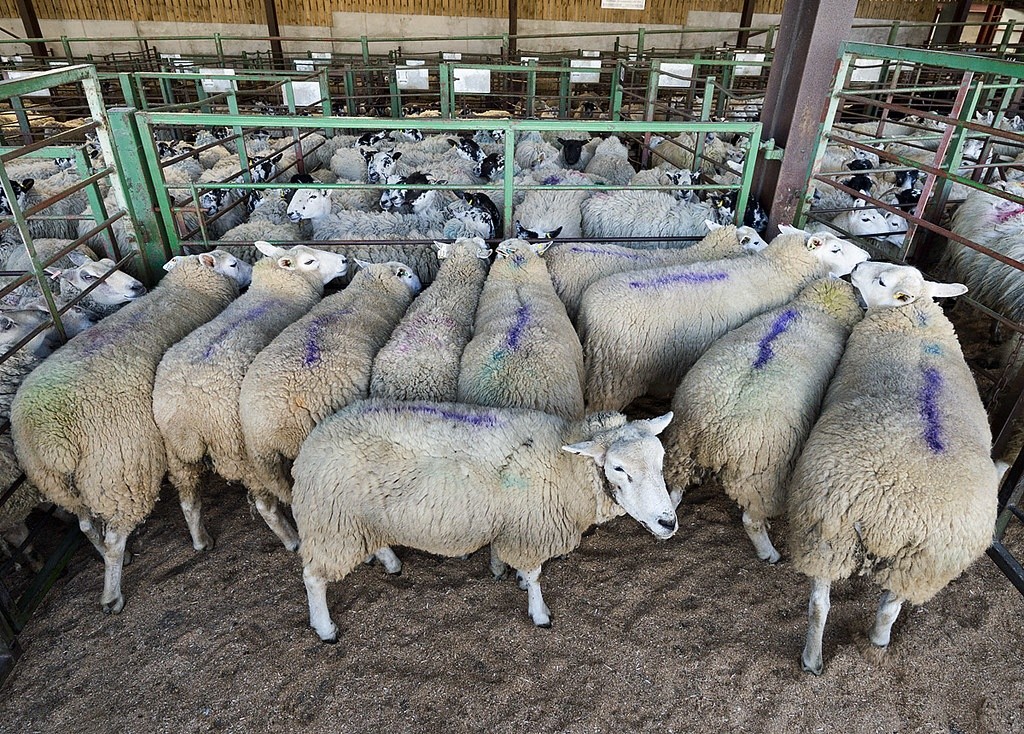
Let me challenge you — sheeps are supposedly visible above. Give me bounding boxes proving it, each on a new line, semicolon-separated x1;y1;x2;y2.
7;249;251;615
238;256;422;551
545;217;769;319
2;92;1024;573
366;236;492;403
153;238;348;550
786;258;1000;677
577;223;872;418
288;396;680;644
453;237;586;419
660;276;867;567
